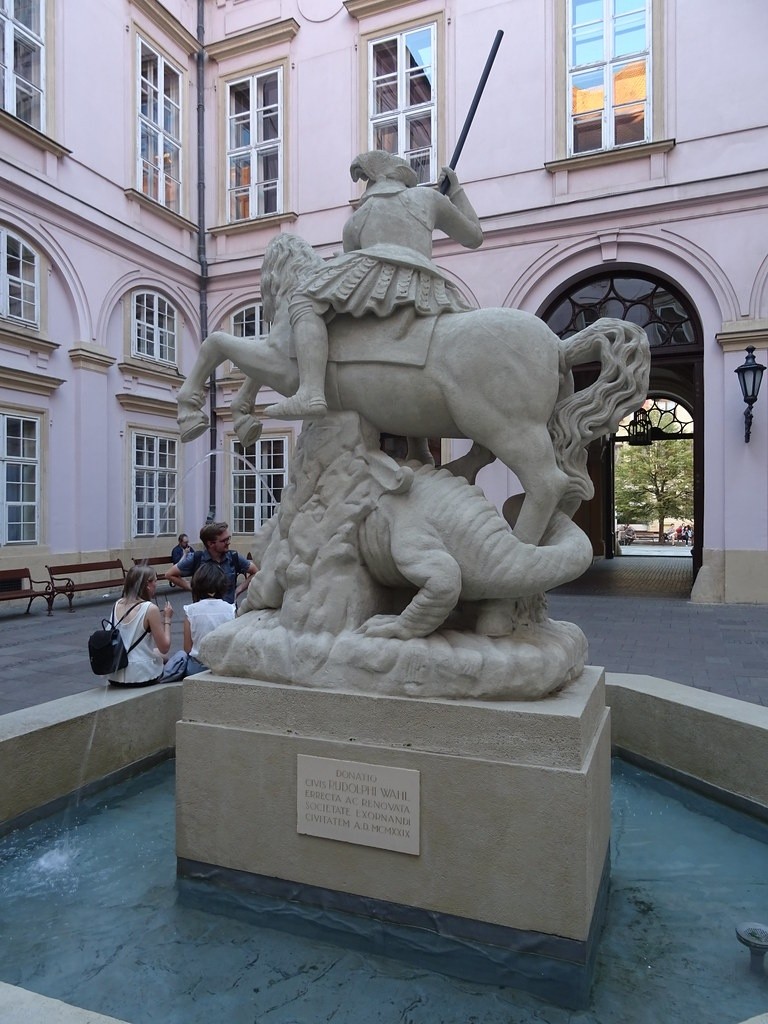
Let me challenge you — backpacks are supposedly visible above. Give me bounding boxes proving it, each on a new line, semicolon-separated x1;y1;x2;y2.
88;601;150;675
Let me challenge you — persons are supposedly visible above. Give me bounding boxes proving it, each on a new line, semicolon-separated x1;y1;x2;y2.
102;566;175;687
164;522;260;678
666;523;694;546
261;150;482;421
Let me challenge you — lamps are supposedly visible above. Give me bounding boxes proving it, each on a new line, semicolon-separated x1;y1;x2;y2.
734;345;767;443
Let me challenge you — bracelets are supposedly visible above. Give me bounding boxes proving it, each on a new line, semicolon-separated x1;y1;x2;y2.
163;623;172;625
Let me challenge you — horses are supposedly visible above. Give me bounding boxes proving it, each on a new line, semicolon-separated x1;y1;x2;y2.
172;232;651;637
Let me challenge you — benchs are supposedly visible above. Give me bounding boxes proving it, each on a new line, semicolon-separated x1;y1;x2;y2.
0;567;55;617
132;556;173;580
633;533;655;544
663;533;694;546
45;558;129;612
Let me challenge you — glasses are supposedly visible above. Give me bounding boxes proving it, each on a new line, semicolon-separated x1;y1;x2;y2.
211;536;231;544
